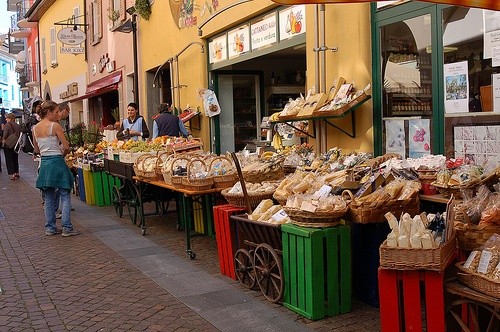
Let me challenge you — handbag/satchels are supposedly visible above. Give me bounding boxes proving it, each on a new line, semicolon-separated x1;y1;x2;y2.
5;134;18;149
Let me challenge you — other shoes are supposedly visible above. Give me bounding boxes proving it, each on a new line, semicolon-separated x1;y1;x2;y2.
11;177;15;180
56;210;62;219
46;230;61;235
15;174;19;178
62;230;80;236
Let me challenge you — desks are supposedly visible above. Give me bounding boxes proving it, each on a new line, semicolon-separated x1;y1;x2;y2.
132;176;224;260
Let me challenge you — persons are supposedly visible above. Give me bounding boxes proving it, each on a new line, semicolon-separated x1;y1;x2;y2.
153;103;188;139
22;120;34;153
2;113;20;180
32;101;80;236
43;103;71;218
120;103;149;140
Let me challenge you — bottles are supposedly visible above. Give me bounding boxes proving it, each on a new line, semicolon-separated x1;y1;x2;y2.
268;68;305;115
384;37;475;113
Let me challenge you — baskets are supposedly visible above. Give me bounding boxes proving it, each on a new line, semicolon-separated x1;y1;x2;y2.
133;149;500;300
279;91;366;122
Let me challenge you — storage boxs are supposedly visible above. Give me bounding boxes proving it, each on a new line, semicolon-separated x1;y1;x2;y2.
75;168;122;207
378;249;474;332
191;193;351;320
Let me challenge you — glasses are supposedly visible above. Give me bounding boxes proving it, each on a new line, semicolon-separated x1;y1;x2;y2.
66;109;71;115
